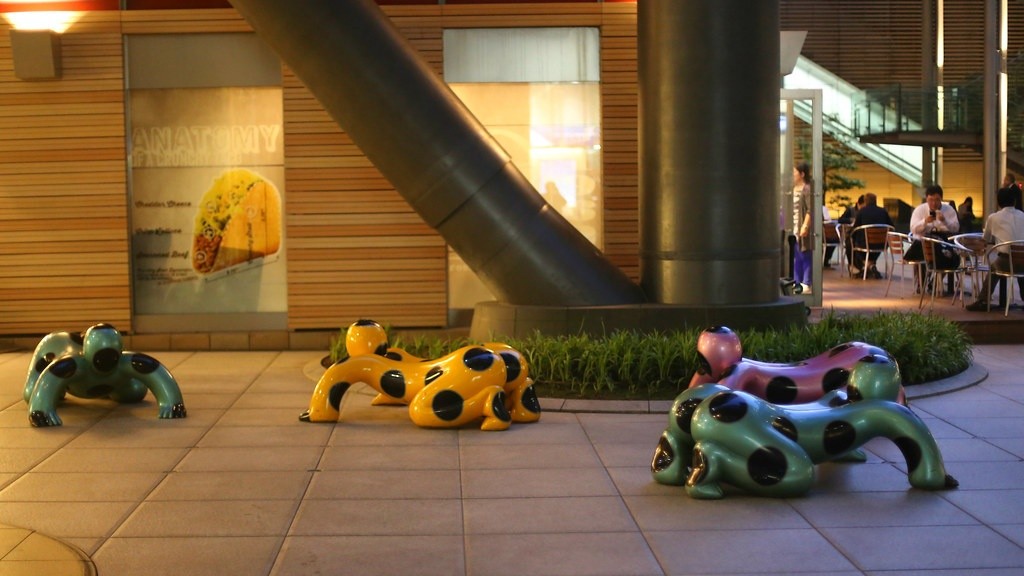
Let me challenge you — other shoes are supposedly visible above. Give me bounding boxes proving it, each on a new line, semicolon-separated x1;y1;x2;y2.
788;252;1024;314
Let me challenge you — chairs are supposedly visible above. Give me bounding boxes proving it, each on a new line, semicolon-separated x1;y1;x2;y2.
985;239;1024;318
849;224;896;281
950;233;995;309
885;231;942;301
919;235;978;312
821;218;855;280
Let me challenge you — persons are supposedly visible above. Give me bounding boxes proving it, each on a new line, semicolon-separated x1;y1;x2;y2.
846;192;894;279
910;185;961;296
793;163;815;295
965;187;1024;312
838;195;866;277
957;198;983;233
1003;174;1022;211
822;205;838;266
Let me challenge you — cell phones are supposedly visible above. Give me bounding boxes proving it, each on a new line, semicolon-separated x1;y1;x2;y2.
930;211;936;220
